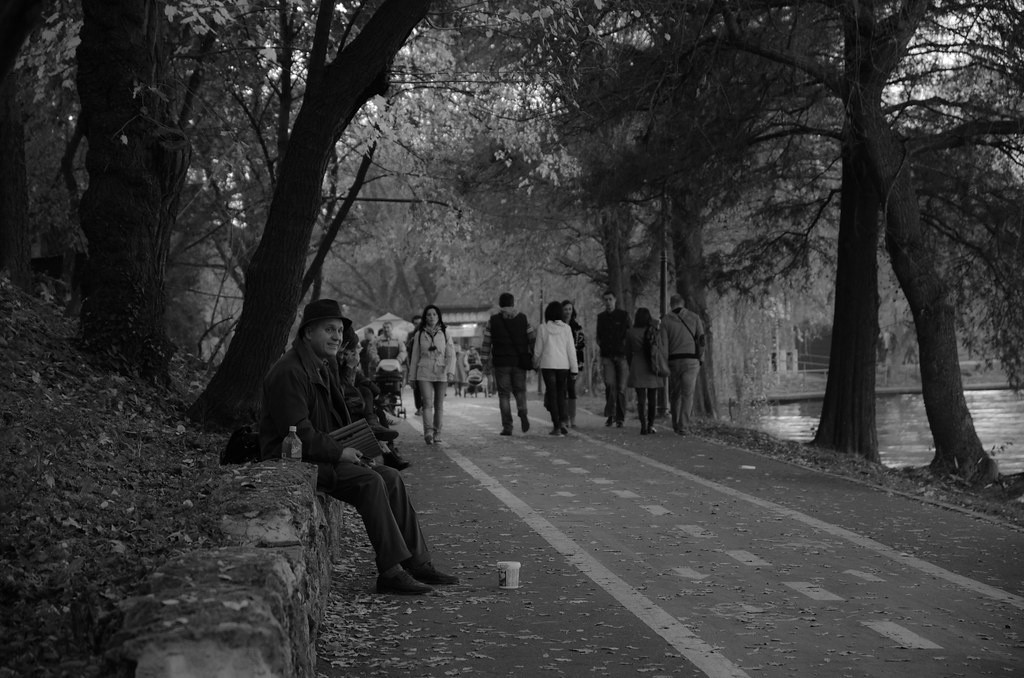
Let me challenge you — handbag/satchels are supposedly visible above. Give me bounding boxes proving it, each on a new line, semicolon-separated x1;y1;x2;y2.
340;385;365;418
651;324;671;377
220;407;262;465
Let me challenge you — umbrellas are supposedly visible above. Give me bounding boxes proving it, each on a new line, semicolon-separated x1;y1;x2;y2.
352;312;415;342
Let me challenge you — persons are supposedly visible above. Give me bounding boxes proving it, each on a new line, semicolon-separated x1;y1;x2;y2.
480;293;537;436
328;316;412;472
661;294;706;435
552;300;585;428
596;292;633;428
262;298;460;595
409;305;456;445
625;308;661;434
532;301;578;436
453;342;497;397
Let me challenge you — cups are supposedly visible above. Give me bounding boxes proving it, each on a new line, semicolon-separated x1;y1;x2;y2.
497;561;521;589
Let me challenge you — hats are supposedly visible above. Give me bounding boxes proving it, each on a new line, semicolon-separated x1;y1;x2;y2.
341;326;359;350
298;299;352;337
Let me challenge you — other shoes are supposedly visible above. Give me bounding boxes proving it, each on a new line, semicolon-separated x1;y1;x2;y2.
415;411;421;415
648;426;656;433
500;430;512;435
433;436;442;443
377;410;401;425
549;429;561;434
605;420;612;425
561;427;568;434
521;419;530;432
641;429;647;434
616;421;623;426
424;433;433;445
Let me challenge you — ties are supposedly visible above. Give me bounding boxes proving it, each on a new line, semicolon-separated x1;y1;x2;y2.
319;365;328;386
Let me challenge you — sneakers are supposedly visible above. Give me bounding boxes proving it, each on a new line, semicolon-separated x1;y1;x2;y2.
413;564;458;584
376;570;433;593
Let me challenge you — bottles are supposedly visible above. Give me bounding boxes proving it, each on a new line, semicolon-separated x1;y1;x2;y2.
282;426;302;474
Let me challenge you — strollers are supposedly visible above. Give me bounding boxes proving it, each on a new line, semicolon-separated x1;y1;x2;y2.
373;359;407;419
463;364;489;399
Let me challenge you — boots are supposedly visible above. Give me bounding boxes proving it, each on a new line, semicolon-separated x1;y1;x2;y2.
367;416;399;441
383;451;409;471
